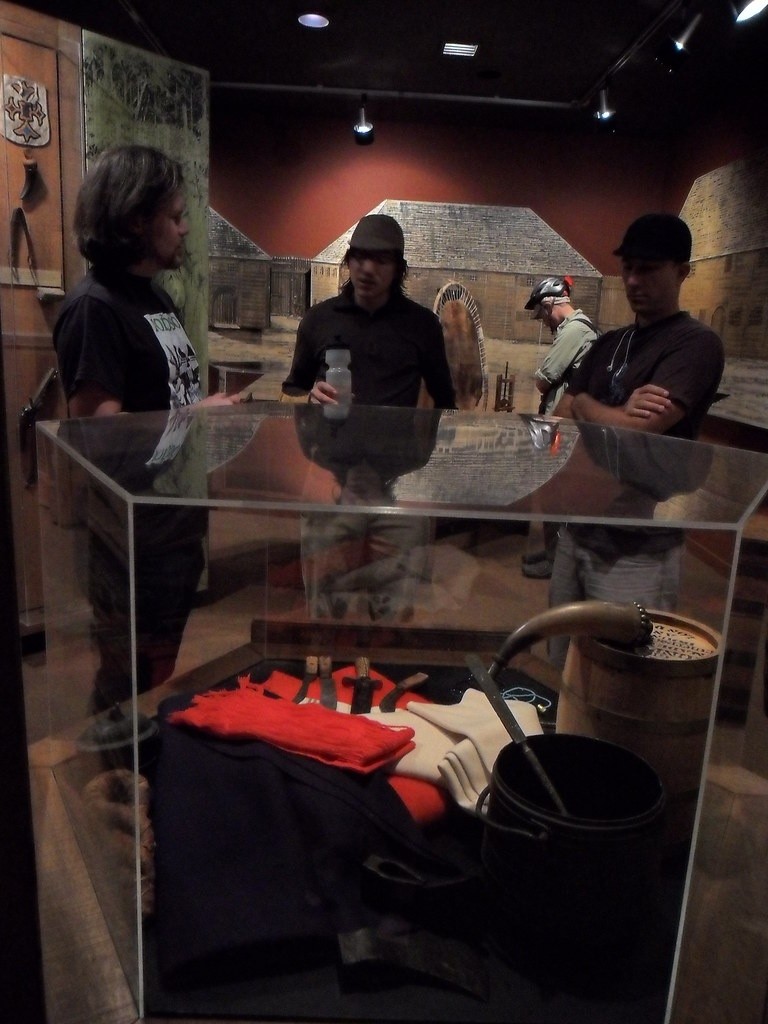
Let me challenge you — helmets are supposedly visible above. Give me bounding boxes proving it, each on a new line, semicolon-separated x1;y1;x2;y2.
523;277;571;310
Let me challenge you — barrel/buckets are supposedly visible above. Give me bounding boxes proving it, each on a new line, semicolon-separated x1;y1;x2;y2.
554;609;722;814
475;735;668;981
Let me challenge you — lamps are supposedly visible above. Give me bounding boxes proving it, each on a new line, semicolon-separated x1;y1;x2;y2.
294;0;337;33
353;122;375;146
730;0;768;24
593;86;618;122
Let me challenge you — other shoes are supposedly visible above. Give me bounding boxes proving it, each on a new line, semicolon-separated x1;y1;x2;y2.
521;550;548;564
522;560;552;578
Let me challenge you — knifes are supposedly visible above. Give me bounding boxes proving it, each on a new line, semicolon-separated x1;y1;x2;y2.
341;657;382;717
317;655;338;711
379;672;430;712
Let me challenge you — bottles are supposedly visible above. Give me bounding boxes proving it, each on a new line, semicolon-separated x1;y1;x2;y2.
323;349;353;421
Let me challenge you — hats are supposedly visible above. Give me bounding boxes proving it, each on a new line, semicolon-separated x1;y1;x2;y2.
530;296;570;320
348;214;404;252
612;213;692;261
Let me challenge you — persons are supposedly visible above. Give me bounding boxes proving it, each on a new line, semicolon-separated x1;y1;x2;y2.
59;410;198;496
522;211;725;673
574;422;715;510
280;212;460;410
52;146;210;720
293;404;444;508
